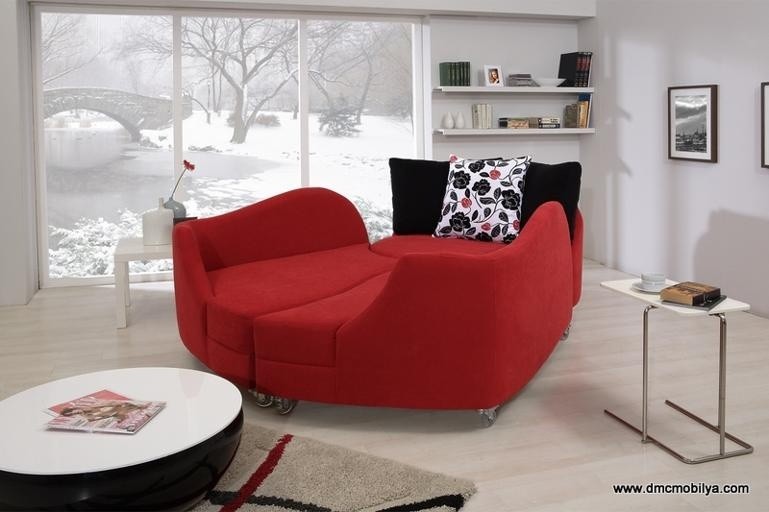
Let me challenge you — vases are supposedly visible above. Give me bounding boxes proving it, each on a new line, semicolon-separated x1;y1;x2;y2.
164;196;186;218
141;197;174;246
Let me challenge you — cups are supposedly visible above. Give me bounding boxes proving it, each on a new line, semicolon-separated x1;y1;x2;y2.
640;272;665;290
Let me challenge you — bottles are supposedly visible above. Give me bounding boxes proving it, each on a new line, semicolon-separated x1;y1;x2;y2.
442;112;465;129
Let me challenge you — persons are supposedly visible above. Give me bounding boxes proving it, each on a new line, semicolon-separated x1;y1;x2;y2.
489;69;498;84
60;399;153;425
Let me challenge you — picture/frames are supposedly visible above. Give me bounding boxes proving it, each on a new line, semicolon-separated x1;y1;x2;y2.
667;85;717;163
760;81;769;167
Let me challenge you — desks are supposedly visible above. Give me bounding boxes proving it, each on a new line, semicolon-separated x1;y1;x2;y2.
599;280;754;465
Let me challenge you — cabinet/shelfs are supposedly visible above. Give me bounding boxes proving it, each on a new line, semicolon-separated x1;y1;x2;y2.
431;85;596;137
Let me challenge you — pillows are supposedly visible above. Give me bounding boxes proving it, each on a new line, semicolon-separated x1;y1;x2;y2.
388;154;582;243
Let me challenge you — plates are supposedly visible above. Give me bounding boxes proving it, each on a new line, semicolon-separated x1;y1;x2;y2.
632;281;674;293
532;78;567;87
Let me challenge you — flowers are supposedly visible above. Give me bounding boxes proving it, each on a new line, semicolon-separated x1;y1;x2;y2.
171;160;195;198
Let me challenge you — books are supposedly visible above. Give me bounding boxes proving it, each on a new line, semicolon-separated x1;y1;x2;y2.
471;102;493;129
498;115;561;129
658;279;721;305
556;51;592;128
660;293;727;312
39;388;130;418
46;398;166;436
439;60;471;86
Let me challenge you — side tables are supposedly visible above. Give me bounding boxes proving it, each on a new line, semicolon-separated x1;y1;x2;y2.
113;237;174;328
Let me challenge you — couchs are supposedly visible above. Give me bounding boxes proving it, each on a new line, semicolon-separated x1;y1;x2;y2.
171;157;584;425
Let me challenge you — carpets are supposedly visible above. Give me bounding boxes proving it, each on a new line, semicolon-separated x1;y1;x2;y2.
188;422;476;512
